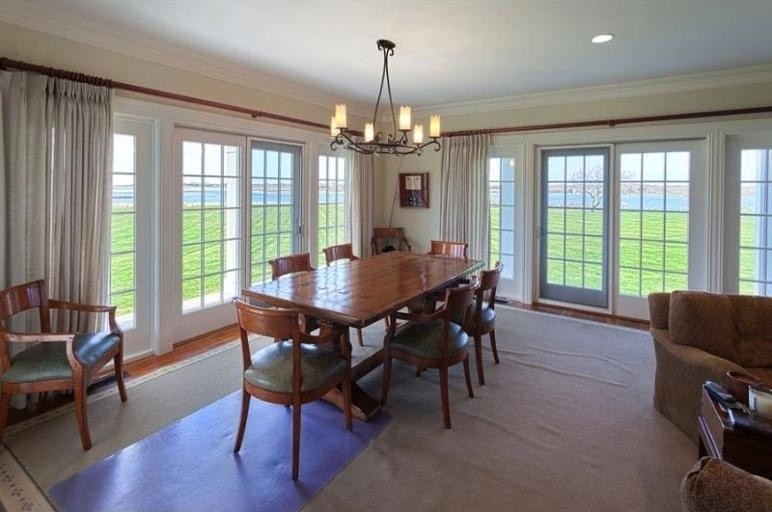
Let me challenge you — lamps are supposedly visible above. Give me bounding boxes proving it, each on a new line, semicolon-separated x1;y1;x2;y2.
330;104;442;157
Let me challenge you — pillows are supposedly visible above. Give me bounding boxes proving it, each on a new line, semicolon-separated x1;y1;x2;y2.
667;289;734;359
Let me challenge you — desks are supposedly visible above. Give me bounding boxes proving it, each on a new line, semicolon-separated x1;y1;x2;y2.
240;251;486;420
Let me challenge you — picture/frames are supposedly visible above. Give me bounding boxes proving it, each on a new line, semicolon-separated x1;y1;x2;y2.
399;172;430;208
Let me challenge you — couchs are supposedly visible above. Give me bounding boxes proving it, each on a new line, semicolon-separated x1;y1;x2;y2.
649;291;772;440
680;456;772;511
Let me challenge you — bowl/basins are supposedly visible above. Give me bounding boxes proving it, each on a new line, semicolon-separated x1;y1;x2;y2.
748;385;772;419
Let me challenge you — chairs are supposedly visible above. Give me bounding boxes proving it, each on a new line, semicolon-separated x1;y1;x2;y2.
1;279;128;450
438;261;505;386
426;239;469;260
324;243;391;348
372;226;411;255
231;298;353;480
376;274;481;429
269;254;316;280
